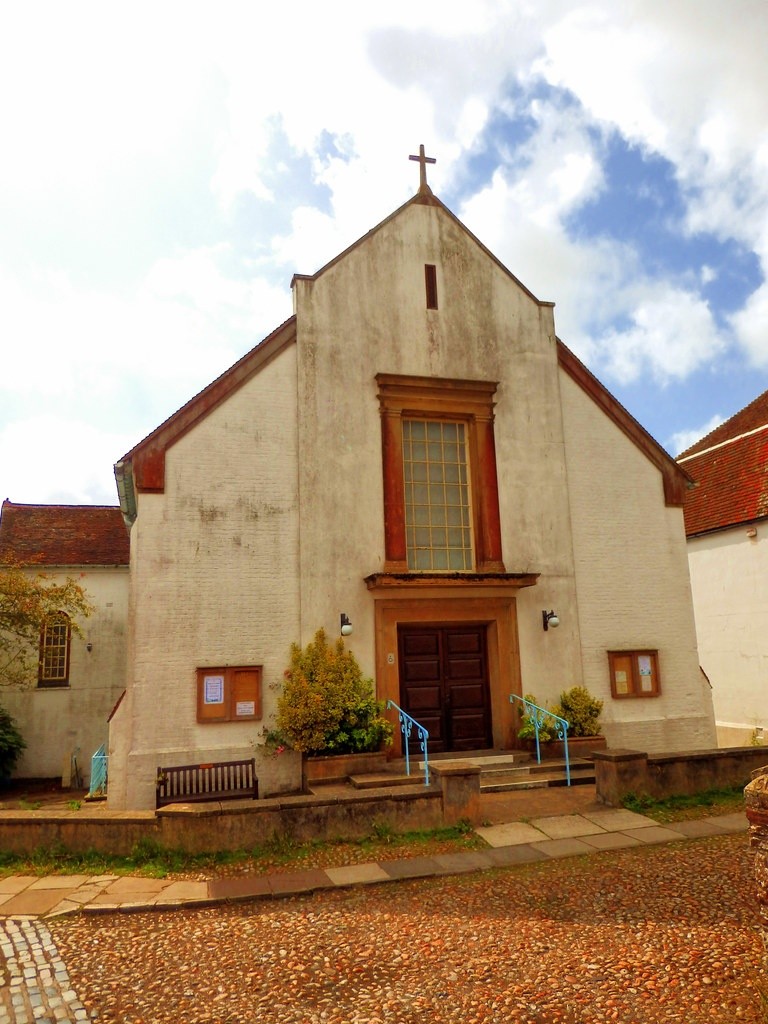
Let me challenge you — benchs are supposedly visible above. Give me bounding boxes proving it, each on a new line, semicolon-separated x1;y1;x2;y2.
155;758;258;808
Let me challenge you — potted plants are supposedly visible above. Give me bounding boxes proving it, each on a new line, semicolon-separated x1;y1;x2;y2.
251;625;396;779
516;686;606;760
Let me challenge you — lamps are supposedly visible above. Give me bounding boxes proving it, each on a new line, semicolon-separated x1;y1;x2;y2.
542;610;560;631
341;614;353;636
